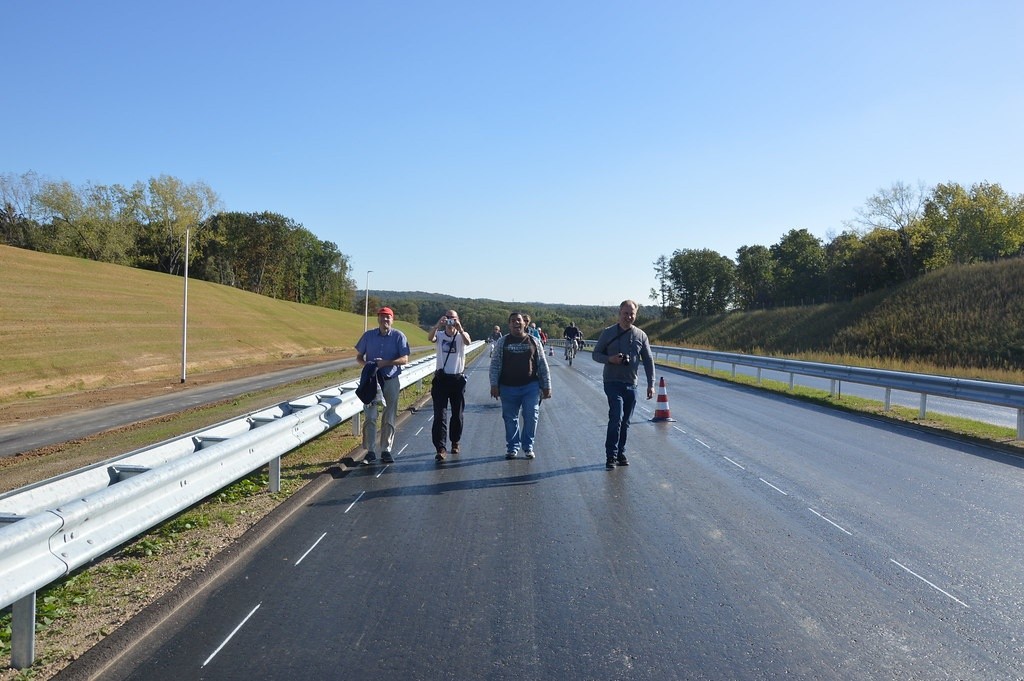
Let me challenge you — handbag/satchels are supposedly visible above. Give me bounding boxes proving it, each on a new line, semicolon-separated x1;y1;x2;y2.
433;369;453;398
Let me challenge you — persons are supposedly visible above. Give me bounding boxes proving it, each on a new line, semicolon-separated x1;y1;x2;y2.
487;325;503;357
354;307;410;465
537;327;546;348
428;310;471;460
592;300;657;471
489;313;552;459
531;322;536;329
522;314;534;336
563;322;580;360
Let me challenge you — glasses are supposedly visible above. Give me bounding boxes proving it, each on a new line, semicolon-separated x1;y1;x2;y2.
524;320;530;323
446;316;455;319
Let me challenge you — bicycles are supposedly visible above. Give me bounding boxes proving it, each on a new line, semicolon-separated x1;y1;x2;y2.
565;336;579;366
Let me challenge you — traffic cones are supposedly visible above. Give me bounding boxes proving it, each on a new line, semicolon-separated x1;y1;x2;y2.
548;344;554;356
648;376;678;422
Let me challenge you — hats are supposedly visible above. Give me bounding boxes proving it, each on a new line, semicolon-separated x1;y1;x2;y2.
377;307;393;317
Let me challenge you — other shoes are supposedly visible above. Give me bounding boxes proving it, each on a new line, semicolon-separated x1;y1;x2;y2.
361;453;376;465
617;457;629;466
506;450;517;459
381;451;394;462
435;447;446;461
525;449;535;458
451;439;460;454
606;458;616;471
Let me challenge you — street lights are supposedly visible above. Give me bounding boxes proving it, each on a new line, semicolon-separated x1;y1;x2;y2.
364;270;373;332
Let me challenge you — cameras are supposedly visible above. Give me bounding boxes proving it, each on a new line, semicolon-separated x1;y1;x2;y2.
619;354;631;365
445;319;455;326
